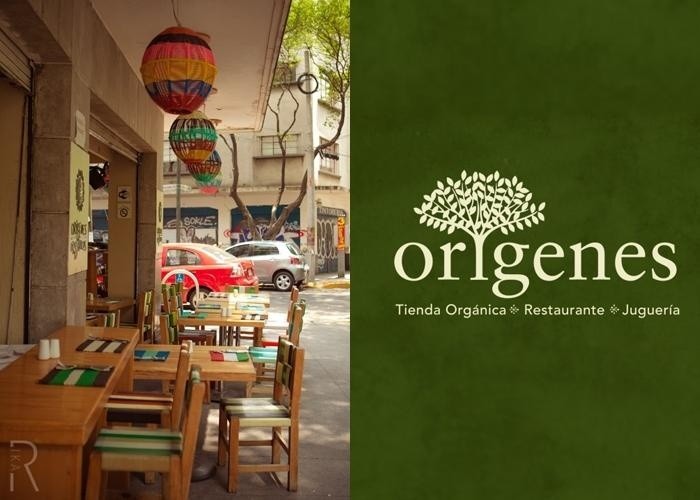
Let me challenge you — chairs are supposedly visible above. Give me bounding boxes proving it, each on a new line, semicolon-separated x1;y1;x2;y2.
82;280;308;500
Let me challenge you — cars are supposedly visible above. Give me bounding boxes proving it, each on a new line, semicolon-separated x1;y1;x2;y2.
89;232;307;312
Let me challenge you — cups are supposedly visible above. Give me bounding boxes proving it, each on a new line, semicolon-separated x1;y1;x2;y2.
87;293;94;301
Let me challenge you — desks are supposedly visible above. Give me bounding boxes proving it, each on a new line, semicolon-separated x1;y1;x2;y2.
0;324;140;499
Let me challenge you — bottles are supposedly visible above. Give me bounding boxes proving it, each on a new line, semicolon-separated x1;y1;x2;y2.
221;306;234;318
39;338;50;361
233;288;239;299
50;339;60;360
229;294;235;305
